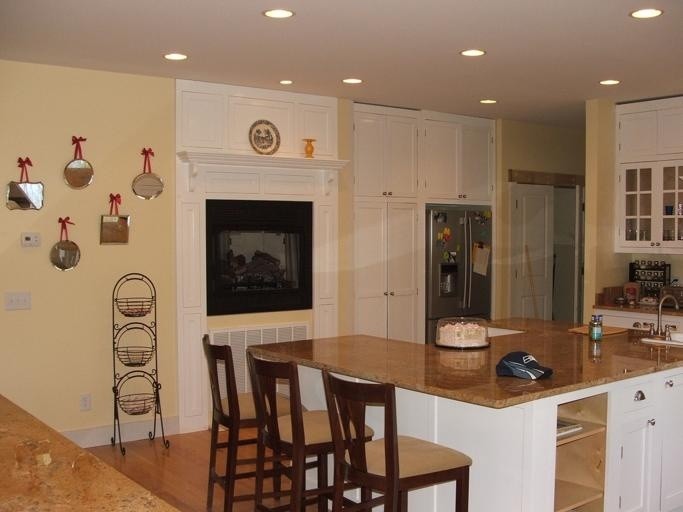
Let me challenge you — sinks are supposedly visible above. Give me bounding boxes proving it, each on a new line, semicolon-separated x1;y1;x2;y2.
640;333;682;347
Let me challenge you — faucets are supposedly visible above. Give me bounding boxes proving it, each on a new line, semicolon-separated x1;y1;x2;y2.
655;294;680;334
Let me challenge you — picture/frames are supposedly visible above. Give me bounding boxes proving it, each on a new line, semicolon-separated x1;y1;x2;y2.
100;215;130;245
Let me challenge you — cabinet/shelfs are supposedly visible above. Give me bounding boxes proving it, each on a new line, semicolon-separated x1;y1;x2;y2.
629;261;671;297
353;103;419;341
543;383;613;512
613;95;683;256
419;109;494;204
613;367;683;511
592;307;683;341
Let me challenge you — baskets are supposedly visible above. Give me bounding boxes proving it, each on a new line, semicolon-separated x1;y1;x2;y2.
118;393;155;415
116;297;153;317
117;346;153;367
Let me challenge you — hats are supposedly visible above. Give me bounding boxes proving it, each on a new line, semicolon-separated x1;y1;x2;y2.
497;352;553;380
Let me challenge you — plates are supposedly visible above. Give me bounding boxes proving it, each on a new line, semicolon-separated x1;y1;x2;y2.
248;120;281;155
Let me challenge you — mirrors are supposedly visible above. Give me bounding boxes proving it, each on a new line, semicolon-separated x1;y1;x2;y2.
5;180;45;210
63;159;94;190
132;172;163;201
50;240;81;272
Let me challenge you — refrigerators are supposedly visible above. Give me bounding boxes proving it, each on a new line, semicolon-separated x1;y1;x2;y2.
426;205;492;350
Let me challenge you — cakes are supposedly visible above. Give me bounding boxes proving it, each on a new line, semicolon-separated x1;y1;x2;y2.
438;323;487;347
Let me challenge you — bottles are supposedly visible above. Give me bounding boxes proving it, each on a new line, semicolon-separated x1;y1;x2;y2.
635;260;665;302
627;229;683;241
588;342;602;365
678;202;683;215
588;321;602;341
590;305;602;327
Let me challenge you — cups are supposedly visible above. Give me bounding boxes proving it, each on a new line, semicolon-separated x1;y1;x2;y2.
665;206;673;215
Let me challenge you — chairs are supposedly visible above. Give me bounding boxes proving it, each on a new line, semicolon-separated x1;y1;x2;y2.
202;333;309;512
321;366;472;512
246;349;374;511
